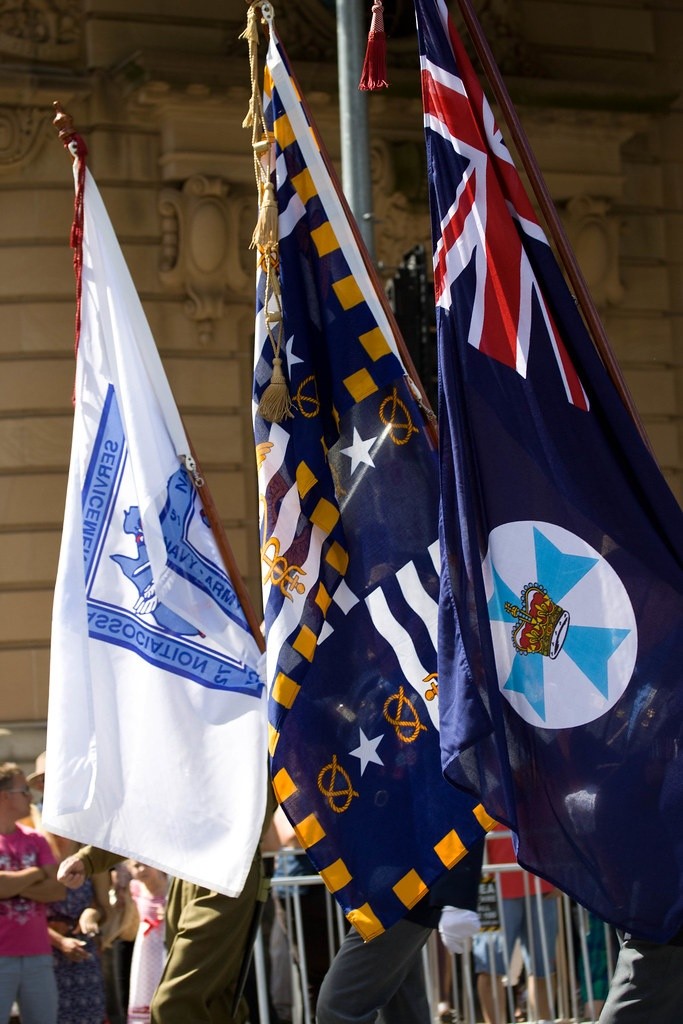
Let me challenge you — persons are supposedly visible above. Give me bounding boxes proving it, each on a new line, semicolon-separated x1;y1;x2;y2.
1;748;683;1024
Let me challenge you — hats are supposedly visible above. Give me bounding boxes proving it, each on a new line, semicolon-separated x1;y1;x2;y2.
26;751;46;790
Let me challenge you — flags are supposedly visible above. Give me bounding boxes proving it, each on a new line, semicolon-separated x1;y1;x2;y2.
356;0;683;945
240;3;486;944
37;98;272;899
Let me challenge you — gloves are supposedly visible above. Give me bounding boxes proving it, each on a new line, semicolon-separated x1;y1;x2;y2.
438;906;482;955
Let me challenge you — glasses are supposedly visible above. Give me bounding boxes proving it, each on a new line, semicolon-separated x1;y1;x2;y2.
7;785;30;796
